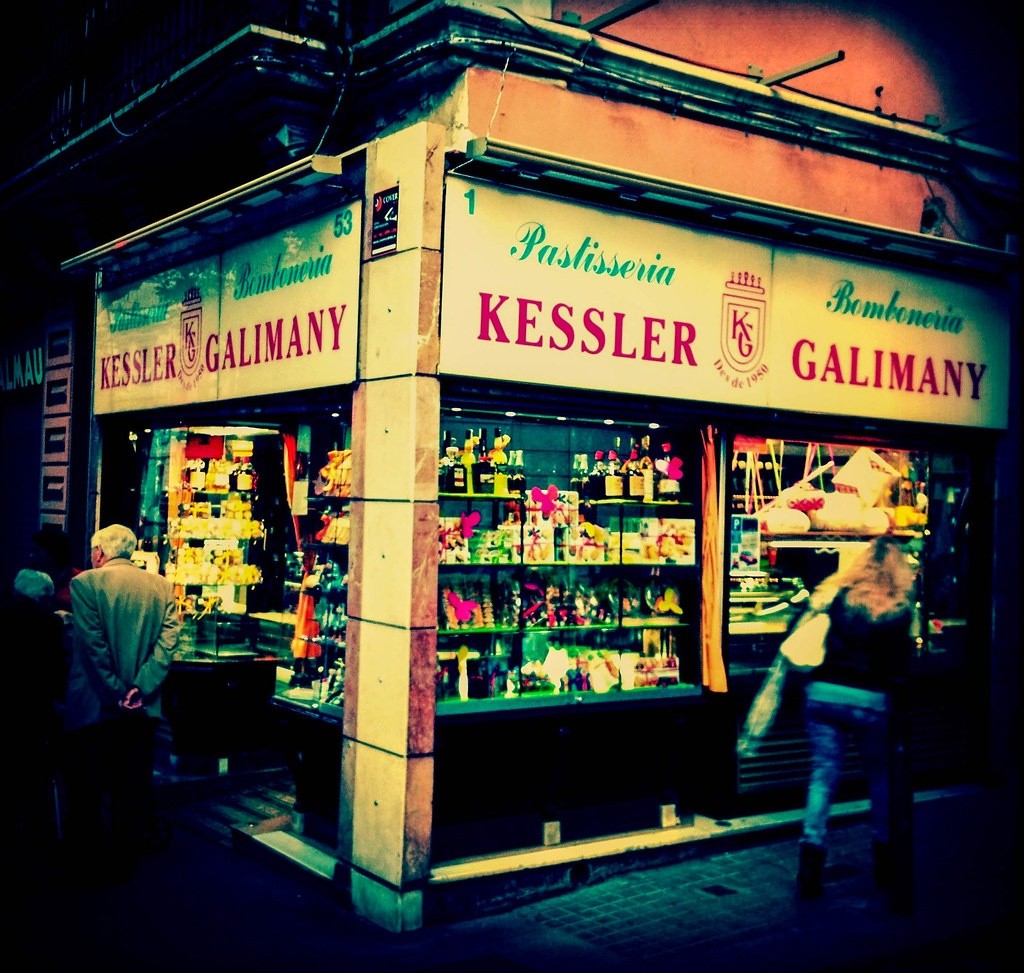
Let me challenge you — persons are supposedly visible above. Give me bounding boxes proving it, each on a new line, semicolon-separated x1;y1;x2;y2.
0;568;73;853
784;535;935;922
64;524;180;888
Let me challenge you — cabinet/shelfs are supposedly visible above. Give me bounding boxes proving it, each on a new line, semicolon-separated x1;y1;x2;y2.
146;423;279;658
727;434;928;648
286;451;350;717
436;402;701;705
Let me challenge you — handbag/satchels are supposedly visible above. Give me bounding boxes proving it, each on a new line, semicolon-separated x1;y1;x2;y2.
777;613;831;673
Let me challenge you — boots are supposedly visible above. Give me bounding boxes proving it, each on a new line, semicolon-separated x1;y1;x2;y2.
795;841;828;902
873;838;890;892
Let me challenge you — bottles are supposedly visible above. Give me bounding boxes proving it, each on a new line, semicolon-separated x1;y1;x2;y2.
571;435;682;500
439;426;527;495
